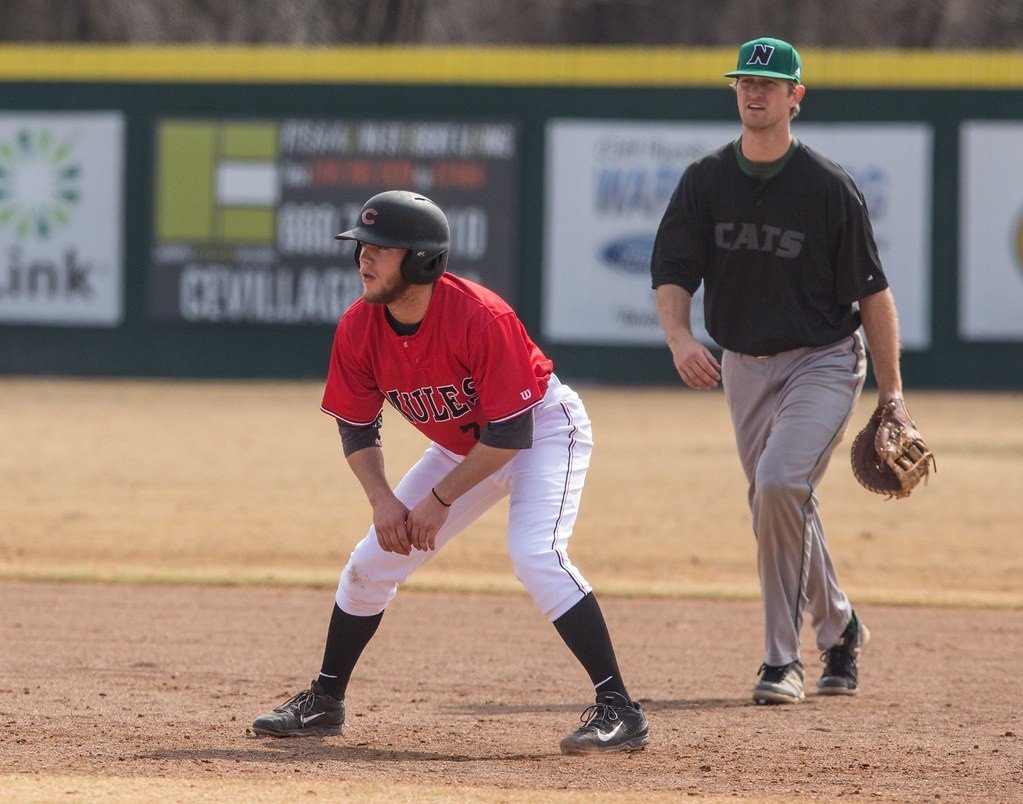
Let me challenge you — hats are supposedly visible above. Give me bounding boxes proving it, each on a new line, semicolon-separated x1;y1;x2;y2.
723;37;801;84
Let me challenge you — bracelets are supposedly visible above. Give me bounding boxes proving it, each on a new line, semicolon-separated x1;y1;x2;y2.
432;488;452;507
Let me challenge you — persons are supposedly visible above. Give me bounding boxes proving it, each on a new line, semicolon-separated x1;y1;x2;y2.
649;37;938;705
246;191;653;754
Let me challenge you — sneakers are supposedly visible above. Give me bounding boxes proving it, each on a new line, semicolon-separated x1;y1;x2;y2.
251;679;346;738
560;690;650;755
816;609;870;696
753;660;806;702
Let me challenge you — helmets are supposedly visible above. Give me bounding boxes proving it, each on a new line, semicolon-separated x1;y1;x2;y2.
335;190;451;285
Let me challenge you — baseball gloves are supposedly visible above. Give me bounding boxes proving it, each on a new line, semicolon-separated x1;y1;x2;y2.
849;396;939;505
